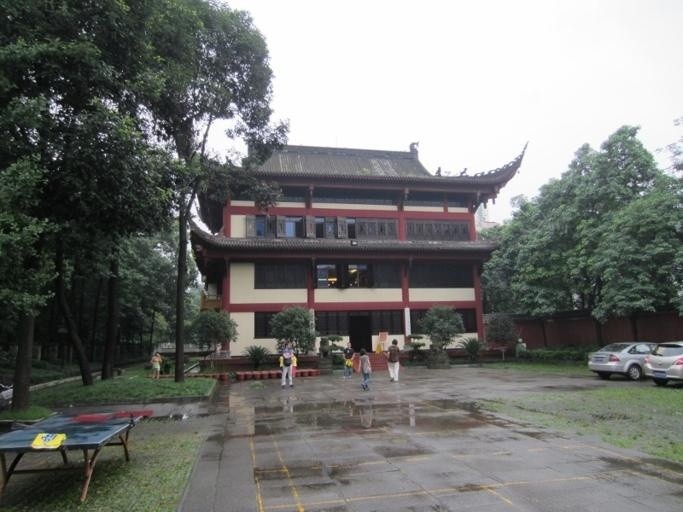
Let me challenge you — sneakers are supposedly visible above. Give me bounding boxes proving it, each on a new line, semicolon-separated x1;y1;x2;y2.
282;376;398;392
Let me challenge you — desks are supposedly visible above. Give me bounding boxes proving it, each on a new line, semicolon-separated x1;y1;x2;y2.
1;409;155;501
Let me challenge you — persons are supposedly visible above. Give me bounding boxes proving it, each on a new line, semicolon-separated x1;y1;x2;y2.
279;355;288;379
281;342;294;388
342;342;356;380
150;351;162;379
358;348;372;391
383;339;399;382
292;352;297;378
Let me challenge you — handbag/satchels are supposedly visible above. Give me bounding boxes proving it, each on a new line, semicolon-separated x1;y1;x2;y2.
363;362;372;374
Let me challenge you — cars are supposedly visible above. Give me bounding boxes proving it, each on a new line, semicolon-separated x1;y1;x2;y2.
644;342;682;384
588;343;658;381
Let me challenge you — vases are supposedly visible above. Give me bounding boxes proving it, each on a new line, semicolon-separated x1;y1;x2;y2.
188;368;320;381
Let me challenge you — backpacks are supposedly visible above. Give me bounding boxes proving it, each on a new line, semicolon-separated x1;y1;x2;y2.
283;355;291;366
389;346;399;362
